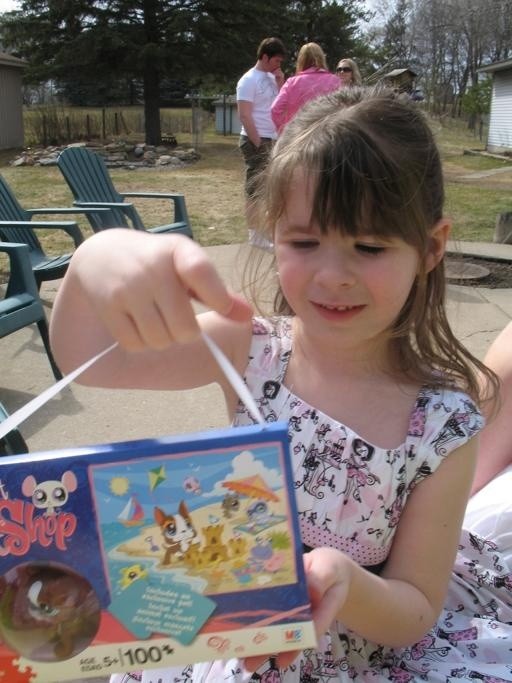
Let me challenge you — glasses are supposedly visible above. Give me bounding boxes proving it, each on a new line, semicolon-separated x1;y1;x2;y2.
335;66;352;73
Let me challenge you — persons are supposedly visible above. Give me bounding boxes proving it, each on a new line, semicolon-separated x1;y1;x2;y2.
462;317;509;565
235;36;362;249
48;86;510;680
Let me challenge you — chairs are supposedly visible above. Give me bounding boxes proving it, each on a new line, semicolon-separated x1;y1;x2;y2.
0;173;122;315
56;146;194;240
0;241;64;457
